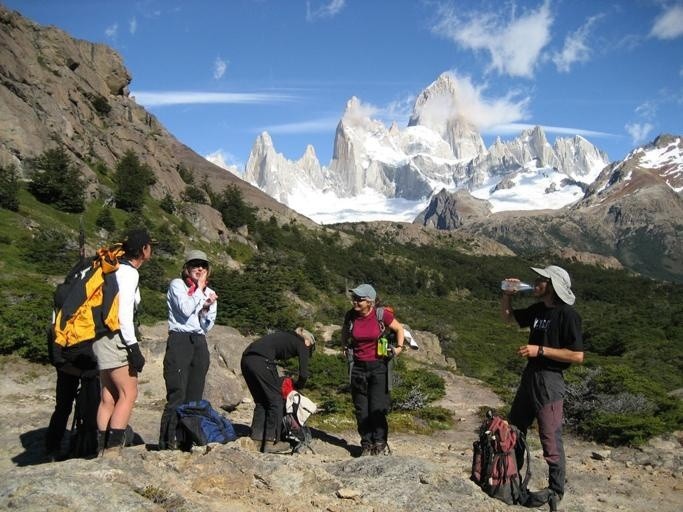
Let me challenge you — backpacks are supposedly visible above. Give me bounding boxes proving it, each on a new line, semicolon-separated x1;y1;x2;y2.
469;412;532;505
176;400;237;446
279;376;312;446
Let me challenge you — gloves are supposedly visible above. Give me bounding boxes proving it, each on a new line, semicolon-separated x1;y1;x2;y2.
127;343;145;372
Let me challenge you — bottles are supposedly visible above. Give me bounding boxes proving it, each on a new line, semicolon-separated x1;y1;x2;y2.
377;336;388;356
500;280;535;292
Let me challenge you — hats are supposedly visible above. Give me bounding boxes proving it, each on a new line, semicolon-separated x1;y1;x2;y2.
348;283;376;299
528;264;575;306
182;250;209;268
124;231;159;250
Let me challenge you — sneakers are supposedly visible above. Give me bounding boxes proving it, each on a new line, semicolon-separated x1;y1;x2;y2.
253;440;290;453
360;439;373;455
534;487;560;502
372;440;389;456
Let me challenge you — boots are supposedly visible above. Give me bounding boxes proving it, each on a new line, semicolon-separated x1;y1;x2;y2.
97;426;136;454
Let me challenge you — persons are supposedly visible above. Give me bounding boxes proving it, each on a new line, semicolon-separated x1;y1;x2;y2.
240;326;315;455
340;282;404;457
159;247;219;449
44;225;151;457
500;264;585;504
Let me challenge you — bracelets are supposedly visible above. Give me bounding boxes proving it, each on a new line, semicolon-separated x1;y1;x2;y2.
396;344;402;349
538;344;542;356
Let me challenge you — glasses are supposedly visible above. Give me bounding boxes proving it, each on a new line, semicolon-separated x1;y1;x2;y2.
351;294;366;301
186;261;209;268
534;276;550;283
